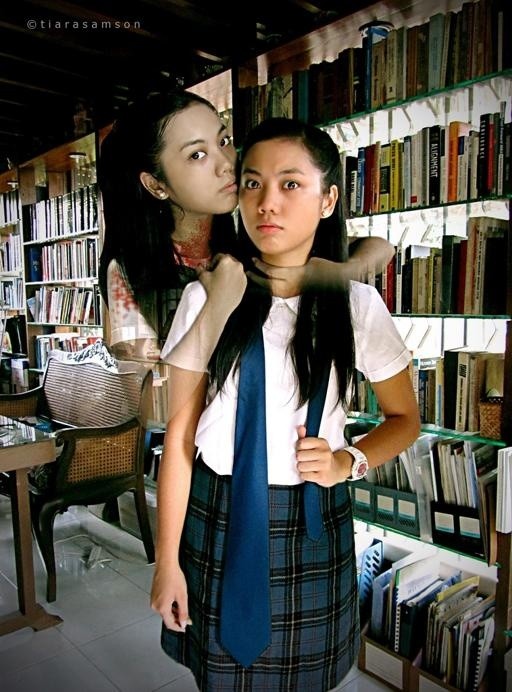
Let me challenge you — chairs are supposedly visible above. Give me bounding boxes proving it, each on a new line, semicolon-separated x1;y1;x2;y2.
1;342;156;602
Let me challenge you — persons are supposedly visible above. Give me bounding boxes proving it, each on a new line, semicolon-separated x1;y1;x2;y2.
97;89;396;432
150;117;420;692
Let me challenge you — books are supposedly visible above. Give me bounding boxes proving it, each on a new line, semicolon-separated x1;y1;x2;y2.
361;216;510;317
0;232;22;272
23;184;103;241
1;277;25;309
23;329;102;372
356;537;495;691
351;433;512;557
344;346;505;439
256;0;510;138
337;100;511;219
0;189;19;228
26;285;99;325
1;314;27;355
24;246;95;281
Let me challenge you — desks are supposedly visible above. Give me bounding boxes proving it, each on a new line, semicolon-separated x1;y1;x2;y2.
0;416;65;637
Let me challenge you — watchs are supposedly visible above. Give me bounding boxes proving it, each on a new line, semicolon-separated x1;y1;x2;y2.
332;446;368;480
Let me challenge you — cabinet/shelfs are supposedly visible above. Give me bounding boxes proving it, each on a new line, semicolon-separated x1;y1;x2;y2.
256;2;511;692
0;67;255;496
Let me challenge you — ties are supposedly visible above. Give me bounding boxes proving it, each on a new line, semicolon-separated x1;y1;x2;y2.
216;335;328;675
